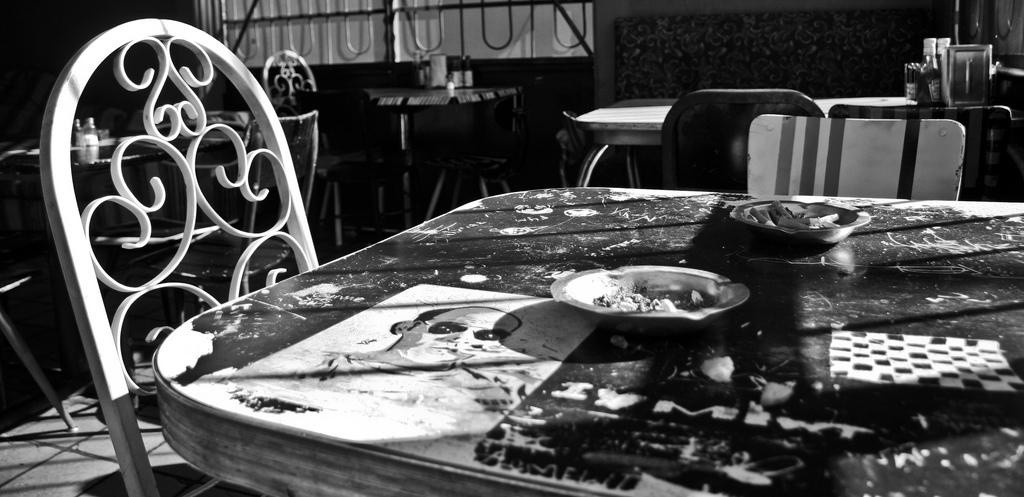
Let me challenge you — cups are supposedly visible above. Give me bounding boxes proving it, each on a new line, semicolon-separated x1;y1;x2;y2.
902;62;920;98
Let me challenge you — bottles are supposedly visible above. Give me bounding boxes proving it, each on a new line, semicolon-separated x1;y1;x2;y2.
72;118;84;147
83;117;99;148
446;73;454;89
463;56;474;88
918;36;940;103
412;50;431;87
448;59;463;88
75;144;100;166
936;37;952;64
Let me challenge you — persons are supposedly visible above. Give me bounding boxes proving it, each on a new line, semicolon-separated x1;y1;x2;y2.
273;304;523;412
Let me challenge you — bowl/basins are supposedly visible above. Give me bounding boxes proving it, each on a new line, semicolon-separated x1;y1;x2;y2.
548;264;750;333
729;198;873;243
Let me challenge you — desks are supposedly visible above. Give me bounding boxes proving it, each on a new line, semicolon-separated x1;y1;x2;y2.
362;84;524;229
572;94;918;199
149;185;1023;496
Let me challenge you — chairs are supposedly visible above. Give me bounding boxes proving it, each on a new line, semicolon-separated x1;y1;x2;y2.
3;0;1024;495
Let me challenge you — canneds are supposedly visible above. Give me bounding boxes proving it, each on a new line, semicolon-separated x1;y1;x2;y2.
429;54;447;89
